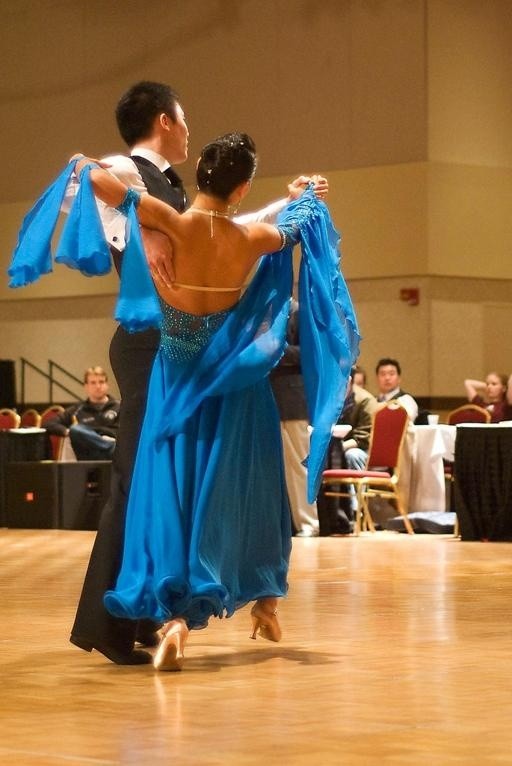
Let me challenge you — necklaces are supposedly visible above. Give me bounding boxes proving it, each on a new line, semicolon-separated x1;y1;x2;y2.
187;205;232;240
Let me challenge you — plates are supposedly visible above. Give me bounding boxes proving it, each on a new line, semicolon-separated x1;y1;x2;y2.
456;419;511;428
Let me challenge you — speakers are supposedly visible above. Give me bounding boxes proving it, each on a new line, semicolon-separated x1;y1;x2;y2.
0;428;48;528
7;460;112;530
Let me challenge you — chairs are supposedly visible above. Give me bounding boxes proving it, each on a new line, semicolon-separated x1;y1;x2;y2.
321;399;415;534
0;405;78;461
443;406;491;511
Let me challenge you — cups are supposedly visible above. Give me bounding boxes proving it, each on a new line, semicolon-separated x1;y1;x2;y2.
427;414;440;427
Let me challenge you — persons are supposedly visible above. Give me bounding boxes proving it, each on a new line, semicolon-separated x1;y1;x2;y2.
45;368;118;462
61;128;334;672
317;366;378;537
462;370;512;424
54;76;192;671
351;366;367;389
343;359;417;523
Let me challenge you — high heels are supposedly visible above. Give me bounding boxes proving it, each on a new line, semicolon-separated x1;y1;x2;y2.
150;617;191;671
248;598;283;643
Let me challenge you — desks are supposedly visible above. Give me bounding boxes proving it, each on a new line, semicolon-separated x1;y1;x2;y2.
453;423;512;541
409;423;457;515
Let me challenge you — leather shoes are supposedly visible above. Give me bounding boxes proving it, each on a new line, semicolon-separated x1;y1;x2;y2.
69;630;153;666
